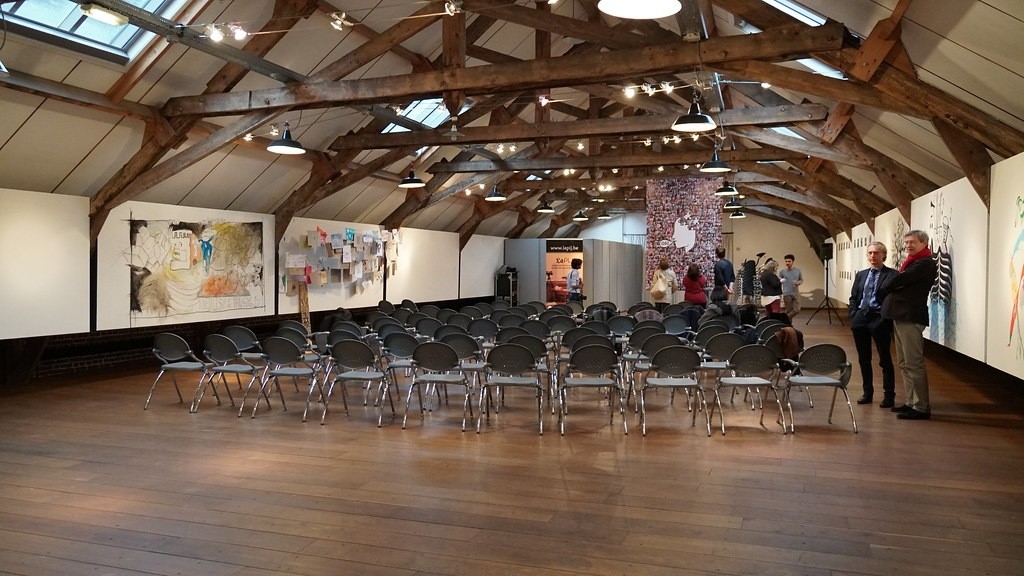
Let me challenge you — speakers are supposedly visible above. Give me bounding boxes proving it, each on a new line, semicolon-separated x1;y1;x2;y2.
820;243;833;260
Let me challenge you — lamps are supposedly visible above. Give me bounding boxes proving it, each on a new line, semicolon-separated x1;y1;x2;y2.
642;83;655;97
698;136;732;173
485;179;506;201
598;210;611;219
227;24;247;41
331;12;346;31
572;211;588;221
714;172;739;195
80;4;128;26
538;201;556;213
266;121;307;154
445;0;463;17
729;209;746;218
670;88;717;133
659;81;674;94
673;135;682;143
538;96;549;107
659;136;670;145
723;172;743;208
442;117;465;141
690;133;699;142
209;24;225;41
398;153;425;188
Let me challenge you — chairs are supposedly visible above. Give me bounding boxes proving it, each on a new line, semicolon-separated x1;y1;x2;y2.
143;299;858;437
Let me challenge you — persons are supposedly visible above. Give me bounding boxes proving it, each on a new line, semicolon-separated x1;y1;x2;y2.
713;246;735;299
653;257;677;311
682;263;708;306
879;230;935;419
779;254;804;324
742;261;756;303
848;242;899;408
760;260;786;315
710;287;728;305
566;259;582;302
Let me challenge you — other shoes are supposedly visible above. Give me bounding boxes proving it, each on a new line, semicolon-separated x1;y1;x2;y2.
892;405;912;412
879;397;896;407
897;409;931;419
857;393;873;404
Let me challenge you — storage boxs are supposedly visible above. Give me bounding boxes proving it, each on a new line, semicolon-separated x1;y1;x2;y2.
496;272;519;308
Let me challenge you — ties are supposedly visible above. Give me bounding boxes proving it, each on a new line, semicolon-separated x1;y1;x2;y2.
861;269;878;316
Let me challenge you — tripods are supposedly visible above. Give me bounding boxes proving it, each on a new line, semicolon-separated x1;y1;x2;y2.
806;260;845;325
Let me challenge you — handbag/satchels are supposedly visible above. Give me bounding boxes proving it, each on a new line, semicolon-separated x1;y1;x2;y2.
569;293;582;301
650;271;668;298
